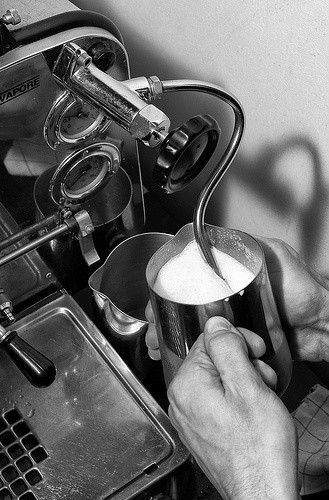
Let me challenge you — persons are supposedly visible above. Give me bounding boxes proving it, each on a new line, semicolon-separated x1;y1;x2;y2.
168;315;299;500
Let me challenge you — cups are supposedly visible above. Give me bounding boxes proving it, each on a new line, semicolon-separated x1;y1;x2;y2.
32;163;136;317
90;233;178;341
145;223;293;399
